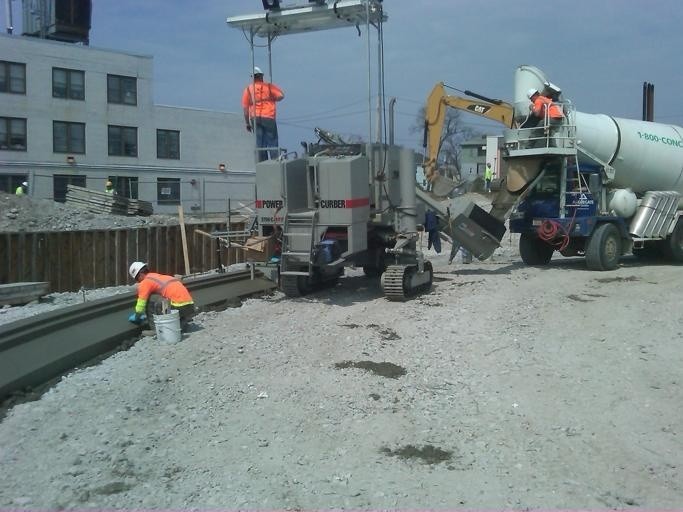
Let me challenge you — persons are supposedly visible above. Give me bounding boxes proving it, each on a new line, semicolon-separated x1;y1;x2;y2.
105;182;117;195
485;163;496;192
16;182;28;196
524;88;564;149
129;262;194;336
242;67;285;162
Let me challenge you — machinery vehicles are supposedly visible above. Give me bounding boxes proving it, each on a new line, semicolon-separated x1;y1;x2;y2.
226;0;435;303
419;79;519;201
497;64;683;272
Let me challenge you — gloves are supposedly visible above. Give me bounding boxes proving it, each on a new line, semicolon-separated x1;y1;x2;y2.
129;314;144;320
245;123;253;133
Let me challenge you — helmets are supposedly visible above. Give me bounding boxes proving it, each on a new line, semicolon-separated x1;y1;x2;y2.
106;182;112;186
249;67;264;77
22;182;27;186
128;262;149;280
526;88;537;100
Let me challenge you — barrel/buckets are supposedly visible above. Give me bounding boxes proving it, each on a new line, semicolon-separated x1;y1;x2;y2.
153;310;181;344
